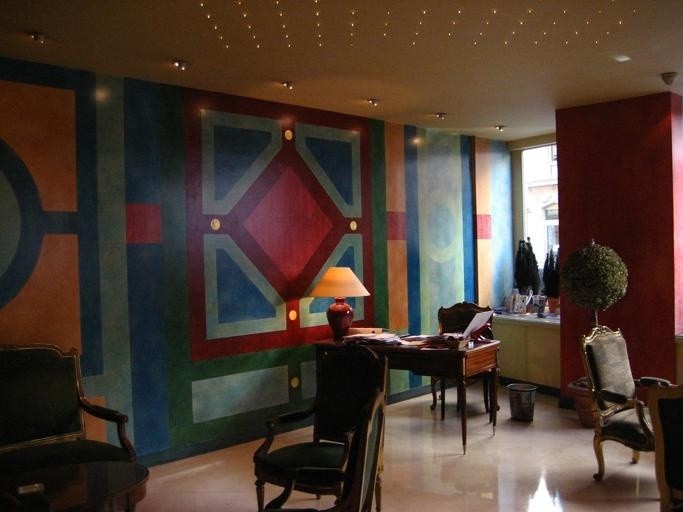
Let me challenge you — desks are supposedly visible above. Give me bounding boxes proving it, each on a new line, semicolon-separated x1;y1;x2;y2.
312;339;502;455
0;462;149;512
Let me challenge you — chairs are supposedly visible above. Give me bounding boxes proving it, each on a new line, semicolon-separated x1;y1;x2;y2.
0;343;149;459
260;390;385;512
579;324;678;481
0;475;53;512
431;300;501;420
252;342;389;512
647;380;683;512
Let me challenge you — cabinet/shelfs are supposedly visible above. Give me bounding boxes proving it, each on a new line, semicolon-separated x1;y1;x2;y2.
493;314;561;388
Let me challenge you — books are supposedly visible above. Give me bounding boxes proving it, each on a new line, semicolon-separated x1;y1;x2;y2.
340;308;496;350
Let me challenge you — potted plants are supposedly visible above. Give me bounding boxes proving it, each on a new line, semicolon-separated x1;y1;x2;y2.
560;242;629;429
514;239;560;312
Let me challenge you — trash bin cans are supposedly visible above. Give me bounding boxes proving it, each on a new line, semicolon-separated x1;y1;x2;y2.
505;383;538;422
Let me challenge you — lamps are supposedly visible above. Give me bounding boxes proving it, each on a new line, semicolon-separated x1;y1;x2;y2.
308;266;372;341
